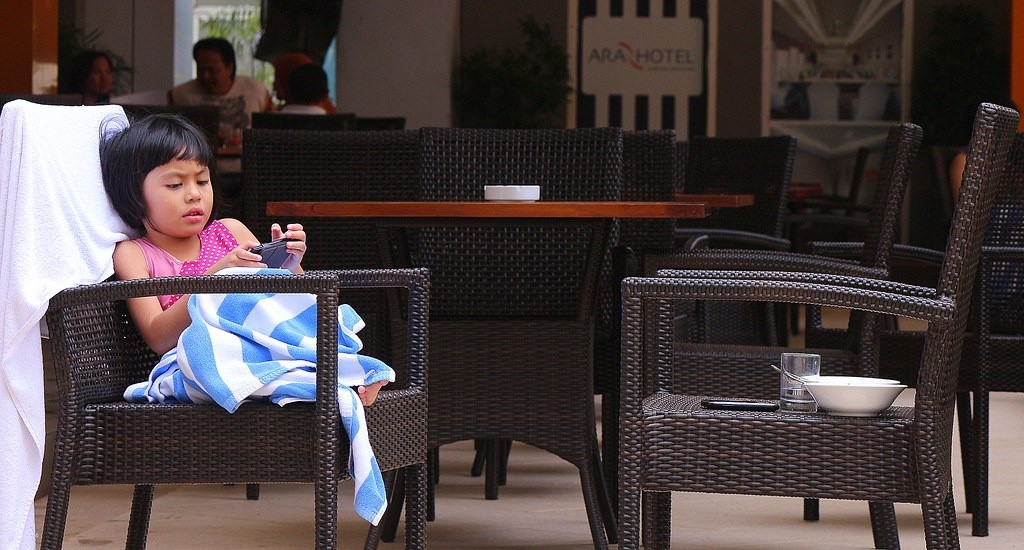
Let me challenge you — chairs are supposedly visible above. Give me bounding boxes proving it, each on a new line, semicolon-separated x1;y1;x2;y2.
0;99;1024;550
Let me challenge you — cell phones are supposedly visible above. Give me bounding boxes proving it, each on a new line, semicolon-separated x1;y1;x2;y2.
702;399;779;413
250;237;300;270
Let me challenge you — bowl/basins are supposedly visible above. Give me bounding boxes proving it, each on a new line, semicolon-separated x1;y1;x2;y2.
799;376;907;418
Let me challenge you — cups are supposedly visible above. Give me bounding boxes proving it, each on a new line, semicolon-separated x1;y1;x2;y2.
781;354;821;414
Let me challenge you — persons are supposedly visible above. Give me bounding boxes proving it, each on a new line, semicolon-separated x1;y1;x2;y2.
949;92;1020;210
274;54;338;115
98;113;389;408
177;37;271;172
68;52;112;105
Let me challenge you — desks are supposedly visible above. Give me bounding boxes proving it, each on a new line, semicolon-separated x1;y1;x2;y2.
266;202;714;550
677;192;754;343
